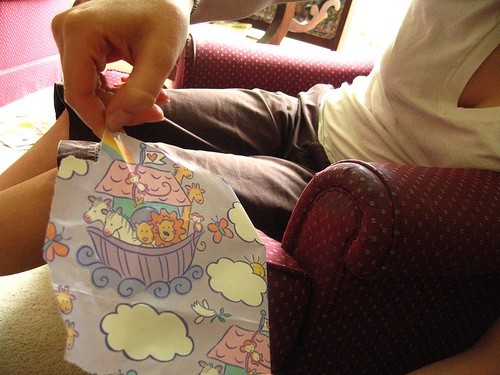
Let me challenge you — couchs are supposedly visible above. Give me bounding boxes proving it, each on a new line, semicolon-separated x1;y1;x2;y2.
174;32;499;375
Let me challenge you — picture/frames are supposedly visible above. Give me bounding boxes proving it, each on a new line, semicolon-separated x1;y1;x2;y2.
234;0;352;51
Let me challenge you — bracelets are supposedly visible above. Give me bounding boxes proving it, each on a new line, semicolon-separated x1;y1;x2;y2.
190;0;199;17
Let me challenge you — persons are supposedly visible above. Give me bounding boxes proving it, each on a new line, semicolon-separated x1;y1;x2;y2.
1;1;499;279
